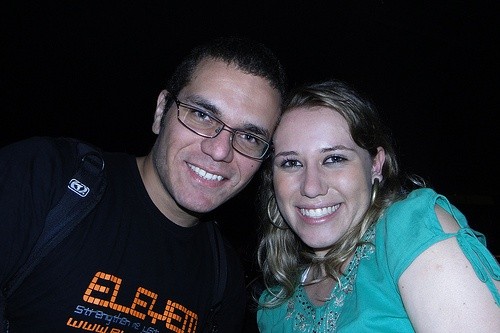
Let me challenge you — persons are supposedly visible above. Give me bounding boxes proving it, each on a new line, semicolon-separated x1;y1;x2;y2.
249;80;500;333
0;34;291;333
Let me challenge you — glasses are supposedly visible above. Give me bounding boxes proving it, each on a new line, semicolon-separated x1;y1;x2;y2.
170;94;273;160
